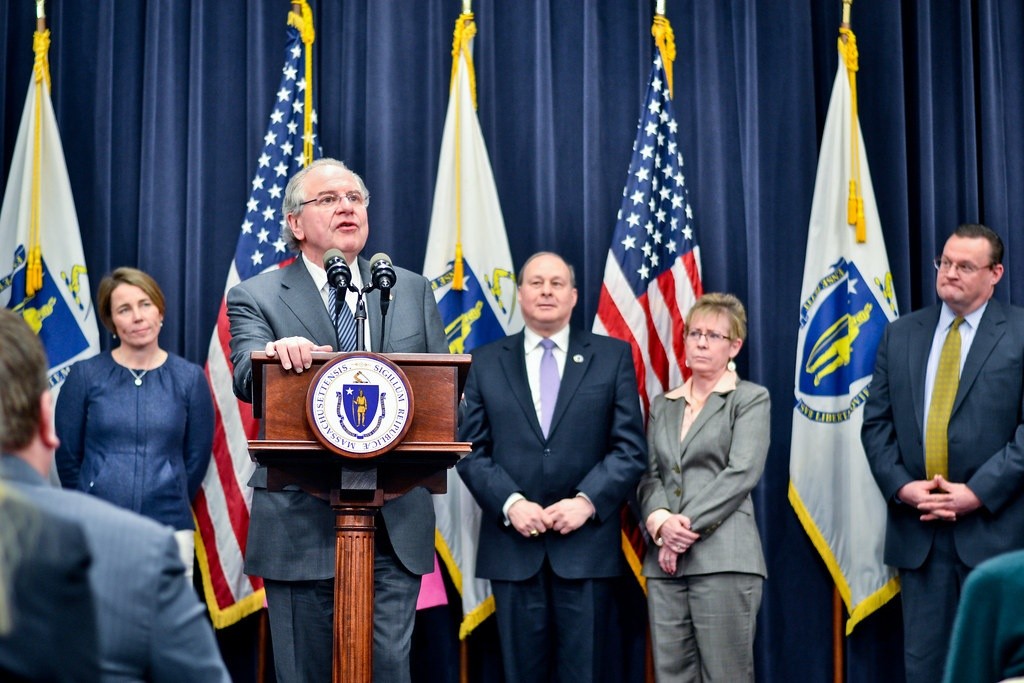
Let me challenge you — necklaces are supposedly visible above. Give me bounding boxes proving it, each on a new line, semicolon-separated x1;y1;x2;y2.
121;358;151;385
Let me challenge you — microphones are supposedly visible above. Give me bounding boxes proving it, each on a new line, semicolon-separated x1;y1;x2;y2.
322;248;352;314
369;252;397;315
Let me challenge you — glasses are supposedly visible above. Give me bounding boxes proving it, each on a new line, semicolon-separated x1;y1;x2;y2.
687;329;733;344
933;257;991;275
295;193;367;210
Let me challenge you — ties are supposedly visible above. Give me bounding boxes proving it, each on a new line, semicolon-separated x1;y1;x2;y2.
329;284;367;352
539;338;560;438
924;316;966;494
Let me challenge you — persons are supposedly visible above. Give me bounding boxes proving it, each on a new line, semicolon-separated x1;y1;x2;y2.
632;293;771;683
227;158;448;683
456;251;647;682
862;225;1024;683
55;267;215;590
0;310;231;683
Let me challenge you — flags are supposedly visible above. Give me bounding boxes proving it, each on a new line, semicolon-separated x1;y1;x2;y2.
423;15;524;639
591;15;702;594
0;30;102;405
788;26;901;634
189;12;324;629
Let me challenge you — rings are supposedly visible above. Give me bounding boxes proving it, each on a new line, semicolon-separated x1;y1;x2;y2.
530;529;537;536
664;558;670;562
